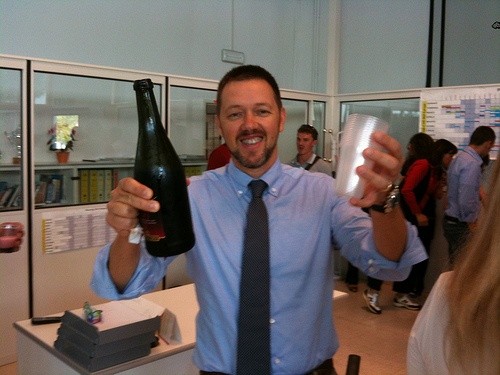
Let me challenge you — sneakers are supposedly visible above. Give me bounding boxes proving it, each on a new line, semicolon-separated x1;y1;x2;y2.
392;293;419;311
362;288;383;314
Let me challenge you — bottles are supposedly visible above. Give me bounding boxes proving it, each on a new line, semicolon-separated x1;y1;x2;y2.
132;78;197;258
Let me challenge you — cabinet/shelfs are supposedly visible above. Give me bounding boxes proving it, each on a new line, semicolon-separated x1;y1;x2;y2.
0;158;208;366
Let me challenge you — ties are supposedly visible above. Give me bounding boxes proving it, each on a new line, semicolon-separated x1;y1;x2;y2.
235;180;274;375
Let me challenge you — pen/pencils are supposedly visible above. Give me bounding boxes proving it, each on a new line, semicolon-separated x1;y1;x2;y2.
31;316;65;324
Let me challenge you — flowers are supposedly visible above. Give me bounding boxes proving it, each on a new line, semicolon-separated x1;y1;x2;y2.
47;124;78;152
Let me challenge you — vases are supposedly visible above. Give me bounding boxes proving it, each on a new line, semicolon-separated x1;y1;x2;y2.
56;152;69;163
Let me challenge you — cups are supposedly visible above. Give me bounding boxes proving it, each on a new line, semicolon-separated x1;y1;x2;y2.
335;112;390;200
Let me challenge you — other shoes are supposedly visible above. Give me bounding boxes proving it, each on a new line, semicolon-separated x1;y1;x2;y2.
346;282;358;291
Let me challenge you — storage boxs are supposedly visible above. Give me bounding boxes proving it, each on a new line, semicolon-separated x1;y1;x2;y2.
54;299;165;372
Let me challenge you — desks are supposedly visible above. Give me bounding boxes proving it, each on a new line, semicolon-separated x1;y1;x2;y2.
13;283;349;375
